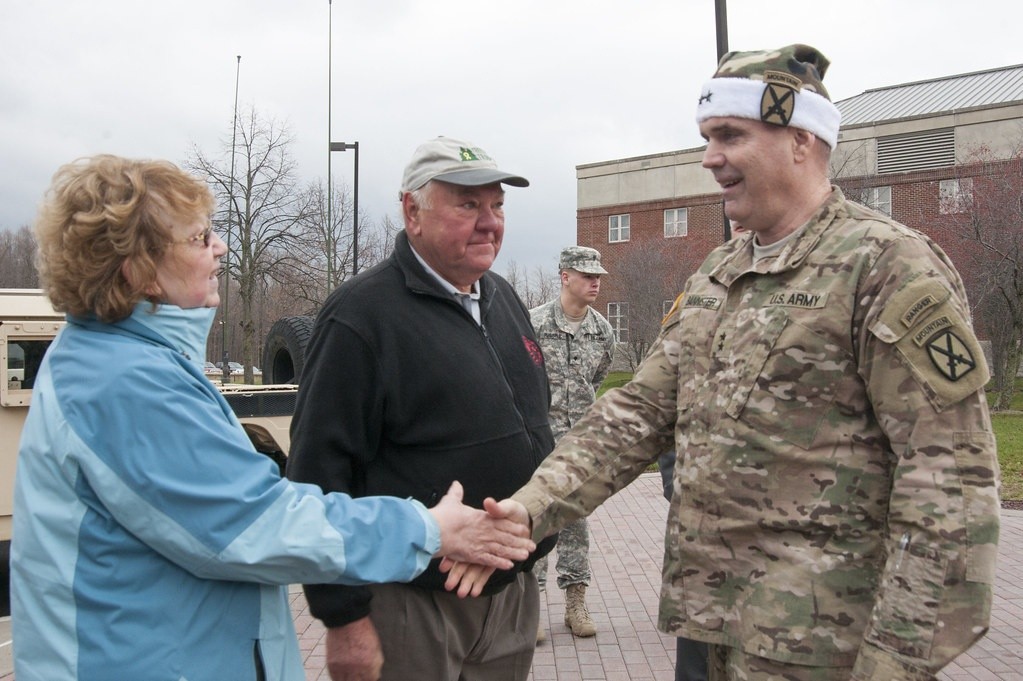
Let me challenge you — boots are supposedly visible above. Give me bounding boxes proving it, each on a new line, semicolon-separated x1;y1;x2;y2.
564;583;599;637
537;625;546;644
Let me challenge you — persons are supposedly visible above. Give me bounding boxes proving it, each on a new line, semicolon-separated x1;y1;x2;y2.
524;246;615;642
656;216;752;681
440;44;999;681
8;152;536;681
284;133;558;681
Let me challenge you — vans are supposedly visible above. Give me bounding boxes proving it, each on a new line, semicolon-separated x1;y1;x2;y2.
216;361;244;375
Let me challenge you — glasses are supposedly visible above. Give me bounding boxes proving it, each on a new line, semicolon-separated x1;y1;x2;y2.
150;225;213;248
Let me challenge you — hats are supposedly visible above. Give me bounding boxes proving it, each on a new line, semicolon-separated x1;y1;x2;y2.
696;44;843;151
558;246;608;275
397;136;530;201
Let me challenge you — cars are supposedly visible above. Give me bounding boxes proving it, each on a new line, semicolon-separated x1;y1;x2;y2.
252;366;263;375
204;361;223;373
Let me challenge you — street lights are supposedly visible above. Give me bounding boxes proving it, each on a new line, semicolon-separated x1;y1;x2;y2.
329;141;360;278
